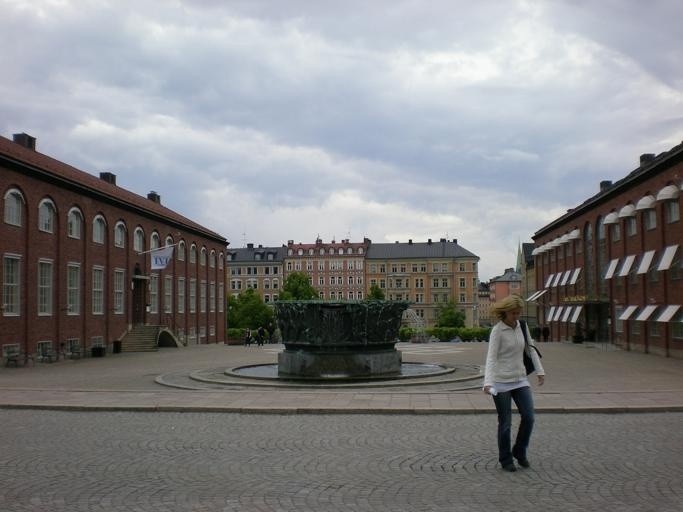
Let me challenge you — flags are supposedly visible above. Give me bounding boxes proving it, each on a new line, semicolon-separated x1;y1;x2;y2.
150;246;173;269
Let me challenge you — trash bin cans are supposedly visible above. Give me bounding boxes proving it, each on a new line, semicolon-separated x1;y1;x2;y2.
91;347;106;357
112;340;121;354
571;336;585;343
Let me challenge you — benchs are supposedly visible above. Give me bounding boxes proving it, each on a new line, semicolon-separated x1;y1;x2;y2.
1;341;93;368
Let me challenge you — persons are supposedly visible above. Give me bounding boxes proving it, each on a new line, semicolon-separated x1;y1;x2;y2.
482;295;545;471
243;321;276;346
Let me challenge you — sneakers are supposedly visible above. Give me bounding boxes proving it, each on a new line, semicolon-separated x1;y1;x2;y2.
502;462;516;472
512;444;529;468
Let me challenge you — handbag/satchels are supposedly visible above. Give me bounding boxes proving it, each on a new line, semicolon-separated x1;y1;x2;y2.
519;320;542;376
